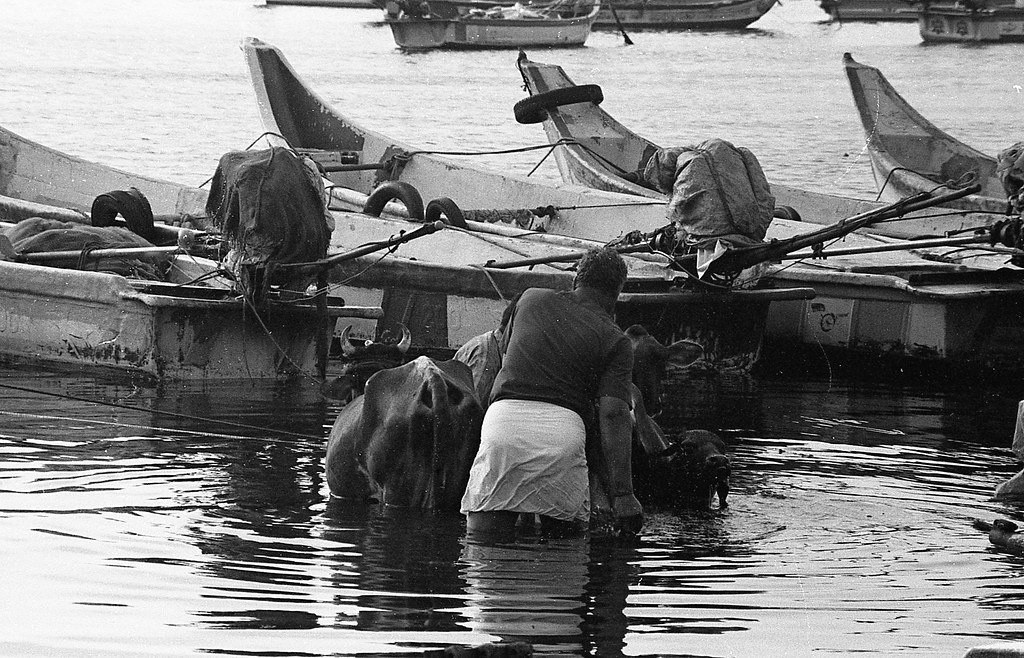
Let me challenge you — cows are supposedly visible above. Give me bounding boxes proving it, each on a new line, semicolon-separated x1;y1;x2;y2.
624;322;733;516
324;322;486;511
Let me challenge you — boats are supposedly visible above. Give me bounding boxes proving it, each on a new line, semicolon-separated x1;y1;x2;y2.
0;1;1024;448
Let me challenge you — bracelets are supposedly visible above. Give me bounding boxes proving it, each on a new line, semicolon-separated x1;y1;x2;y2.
612;491;636;496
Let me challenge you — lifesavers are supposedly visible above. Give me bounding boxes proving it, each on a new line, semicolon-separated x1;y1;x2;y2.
426;196;466;228
513;83;603;125
773;205;801;221
362;180;424;220
91;187;154;239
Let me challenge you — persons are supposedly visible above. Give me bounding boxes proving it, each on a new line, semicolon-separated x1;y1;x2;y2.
461;248;644;537
